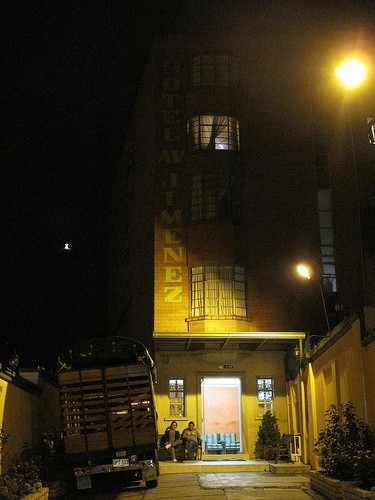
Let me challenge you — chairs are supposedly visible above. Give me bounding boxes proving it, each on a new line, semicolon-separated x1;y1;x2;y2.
204;433;241;454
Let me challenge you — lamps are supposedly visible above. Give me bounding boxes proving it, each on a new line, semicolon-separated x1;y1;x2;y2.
292;344;301;361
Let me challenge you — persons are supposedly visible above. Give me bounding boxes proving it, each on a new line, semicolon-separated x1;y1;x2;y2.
164;421;182;463
181;421;202;459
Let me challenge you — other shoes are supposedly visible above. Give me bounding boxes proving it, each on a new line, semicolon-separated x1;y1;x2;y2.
173;458;178;463
165;444;170;449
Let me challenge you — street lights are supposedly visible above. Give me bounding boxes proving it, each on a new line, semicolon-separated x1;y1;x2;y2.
298;262;330;332
336;56;368;305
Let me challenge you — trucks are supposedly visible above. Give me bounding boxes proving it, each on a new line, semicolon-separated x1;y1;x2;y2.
55;337;159;489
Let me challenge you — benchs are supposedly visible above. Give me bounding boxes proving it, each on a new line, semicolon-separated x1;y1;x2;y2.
156;435;202;461
